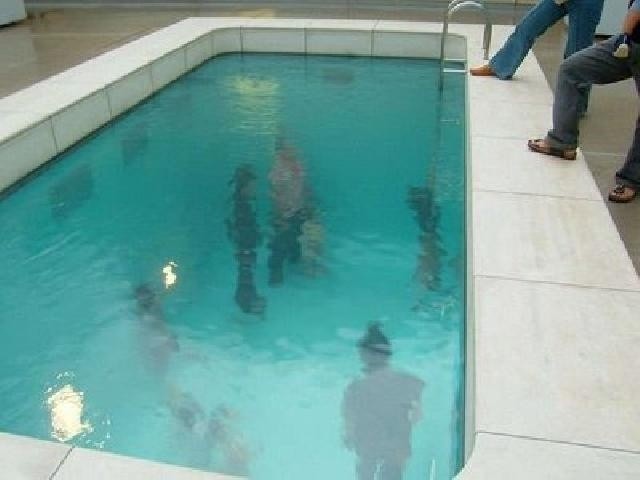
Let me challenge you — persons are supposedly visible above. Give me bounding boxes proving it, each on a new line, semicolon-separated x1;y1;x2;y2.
527;0;640;203
468;1;605;119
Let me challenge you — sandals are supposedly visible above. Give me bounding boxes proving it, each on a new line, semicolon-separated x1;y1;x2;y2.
608;175;638;203
527;135;577;161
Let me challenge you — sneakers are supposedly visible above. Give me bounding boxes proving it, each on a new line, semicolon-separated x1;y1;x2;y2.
611;32;632;60
470;61;495;77
